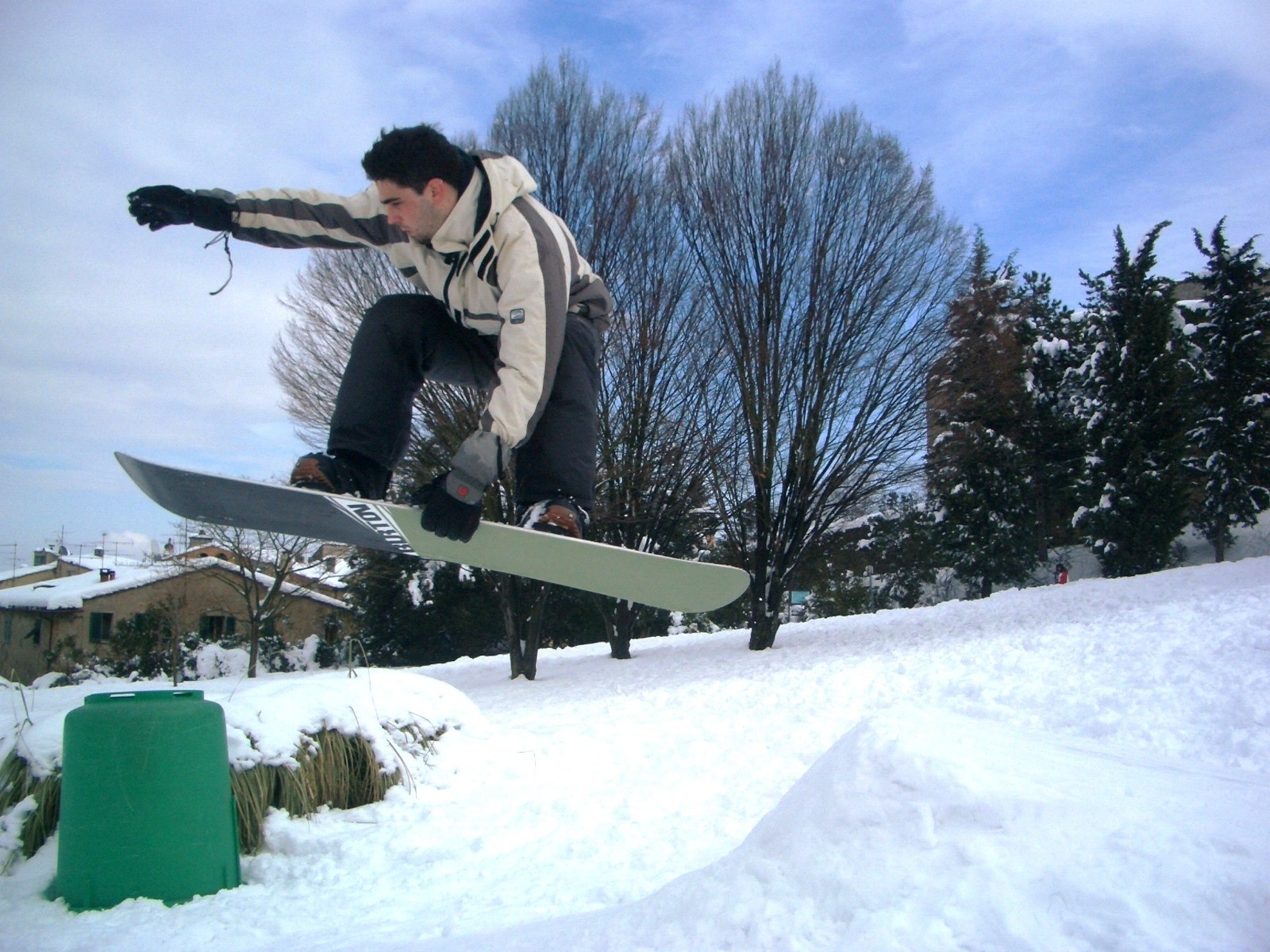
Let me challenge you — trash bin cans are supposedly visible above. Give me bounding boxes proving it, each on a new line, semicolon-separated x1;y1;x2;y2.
43;691;241;913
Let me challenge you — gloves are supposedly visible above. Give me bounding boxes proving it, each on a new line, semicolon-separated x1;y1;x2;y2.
127;186;233;234
407;429;512;541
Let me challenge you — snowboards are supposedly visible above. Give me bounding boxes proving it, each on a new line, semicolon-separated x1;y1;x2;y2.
110;448;753;615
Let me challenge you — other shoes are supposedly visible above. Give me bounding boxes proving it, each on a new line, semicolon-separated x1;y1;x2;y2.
290;454;390;502
517;493;582;537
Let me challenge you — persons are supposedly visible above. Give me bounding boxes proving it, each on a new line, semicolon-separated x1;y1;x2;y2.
128;124;615;544
1056;564;1069;584
